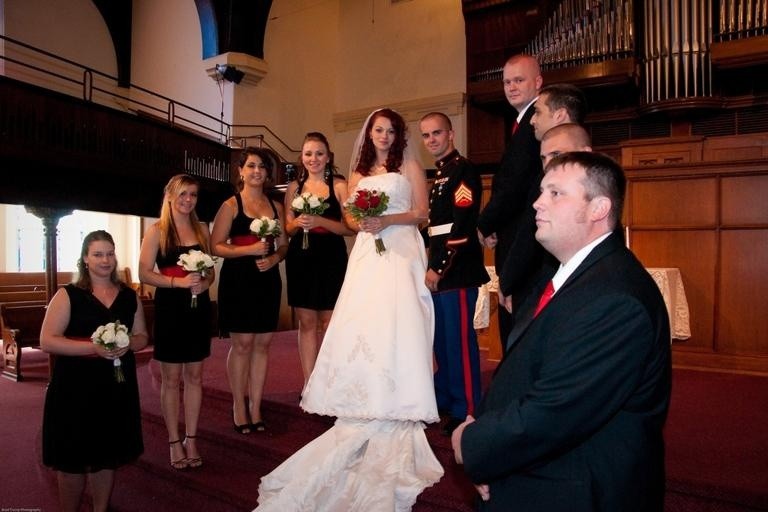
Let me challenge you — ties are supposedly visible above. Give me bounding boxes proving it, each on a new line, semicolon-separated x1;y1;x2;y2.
530;278;555;322
511;119;519;136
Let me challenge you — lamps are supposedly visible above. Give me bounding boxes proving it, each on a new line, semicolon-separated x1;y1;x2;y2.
216;63;246;84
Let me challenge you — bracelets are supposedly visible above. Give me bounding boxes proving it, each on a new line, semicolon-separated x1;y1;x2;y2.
169;276;175;288
358;223;363;231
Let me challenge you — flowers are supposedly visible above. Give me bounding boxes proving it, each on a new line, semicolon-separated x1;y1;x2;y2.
342;188;389;256
176;249;219;308
249;216;283;259
289;191;330;249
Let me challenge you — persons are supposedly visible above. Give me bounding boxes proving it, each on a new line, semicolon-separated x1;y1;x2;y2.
473;54;544;357
38;229;149;512
138;173;215;471
282;131;355;402
538;122;591;170
210;144;289;436
495;83;588;329
451;150;672;511
418;112;490;437
298;106;441;424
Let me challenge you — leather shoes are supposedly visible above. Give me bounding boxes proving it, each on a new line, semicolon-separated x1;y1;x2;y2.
439;416;465;437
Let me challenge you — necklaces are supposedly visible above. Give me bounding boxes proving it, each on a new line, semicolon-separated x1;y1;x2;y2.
373;161;387;171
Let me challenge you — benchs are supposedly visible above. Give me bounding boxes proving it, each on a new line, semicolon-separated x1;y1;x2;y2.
0;267;154;383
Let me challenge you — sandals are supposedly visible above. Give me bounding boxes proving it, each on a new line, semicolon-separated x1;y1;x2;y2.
168;437;187;469
182;436;203;468
247;404;267;433
231;404;252;434
